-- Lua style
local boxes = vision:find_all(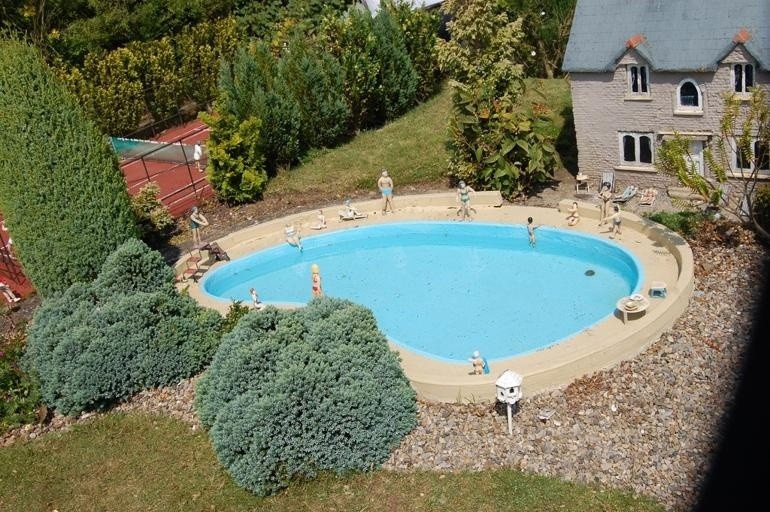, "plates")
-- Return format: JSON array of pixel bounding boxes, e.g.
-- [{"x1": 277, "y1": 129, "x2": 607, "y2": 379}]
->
[{"x1": 630, "y1": 294, "x2": 643, "y2": 301}]
